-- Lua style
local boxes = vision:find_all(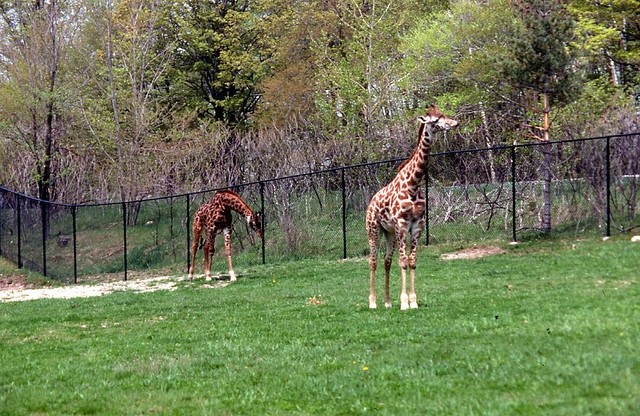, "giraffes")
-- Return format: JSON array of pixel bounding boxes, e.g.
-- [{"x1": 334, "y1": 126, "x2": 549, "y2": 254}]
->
[
  {"x1": 185, "y1": 188, "x2": 263, "y2": 284},
  {"x1": 363, "y1": 103, "x2": 461, "y2": 311}
]
